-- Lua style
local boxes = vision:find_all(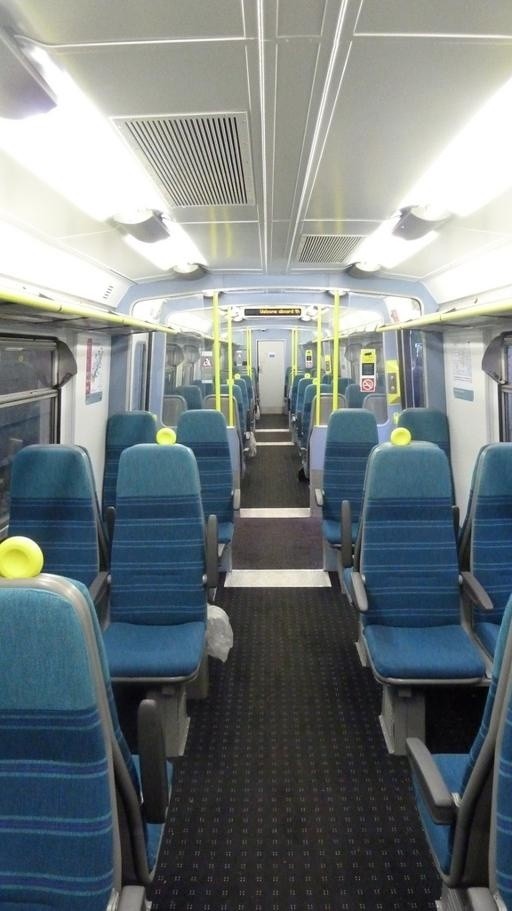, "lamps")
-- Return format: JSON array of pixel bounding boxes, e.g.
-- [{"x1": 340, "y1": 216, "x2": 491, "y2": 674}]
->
[{"x1": 344, "y1": 206, "x2": 447, "y2": 280}]
